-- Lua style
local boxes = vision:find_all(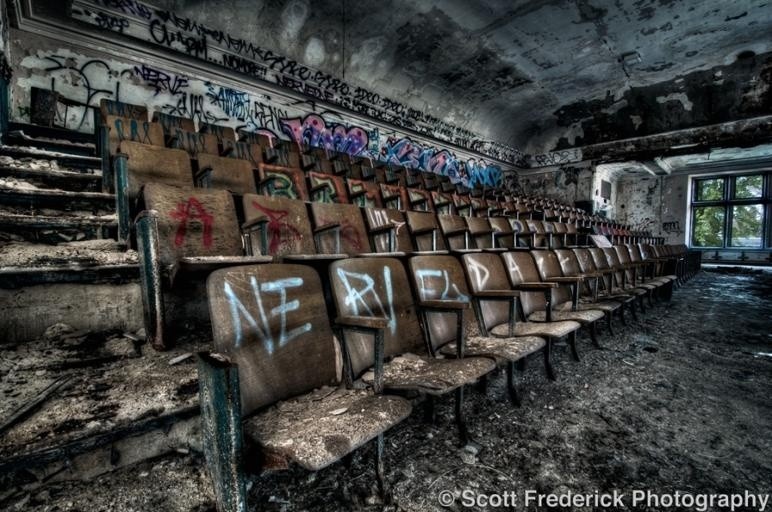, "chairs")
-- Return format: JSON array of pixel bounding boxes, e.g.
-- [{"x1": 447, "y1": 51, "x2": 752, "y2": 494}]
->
[{"x1": 98, "y1": 97, "x2": 690, "y2": 511}]
